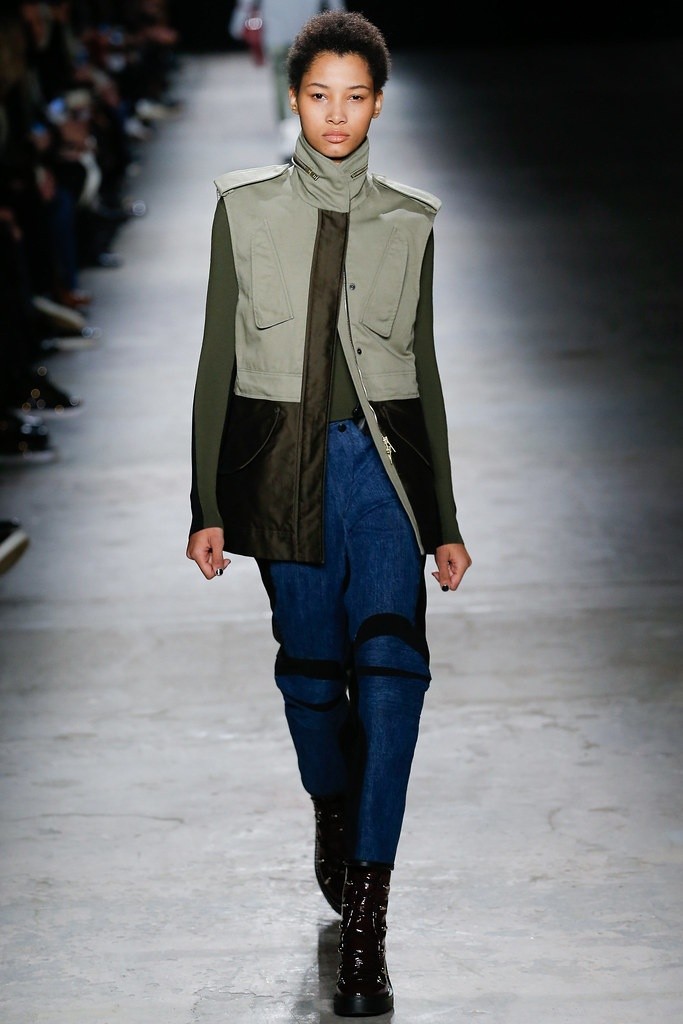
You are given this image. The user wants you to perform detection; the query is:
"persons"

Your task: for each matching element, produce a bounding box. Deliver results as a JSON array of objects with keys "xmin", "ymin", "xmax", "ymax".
[
  {"xmin": 186, "ymin": 8, "xmax": 471, "ymax": 1024},
  {"xmin": 0, "ymin": 0, "xmax": 247, "ymax": 575},
  {"xmin": 231, "ymin": 0, "xmax": 346, "ymax": 132}
]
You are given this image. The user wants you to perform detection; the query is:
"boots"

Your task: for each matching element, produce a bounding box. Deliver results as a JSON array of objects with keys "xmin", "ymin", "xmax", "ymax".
[
  {"xmin": 334, "ymin": 866, "xmax": 392, "ymax": 1016},
  {"xmin": 313, "ymin": 797, "xmax": 355, "ymax": 915}
]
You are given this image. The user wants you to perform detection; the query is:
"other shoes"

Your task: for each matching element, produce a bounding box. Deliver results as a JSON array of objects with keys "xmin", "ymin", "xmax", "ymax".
[
  {"xmin": 0, "ymin": 416, "xmax": 54, "ymax": 464},
  {"xmin": 0, "ymin": 520, "xmax": 30, "ymax": 579}
]
